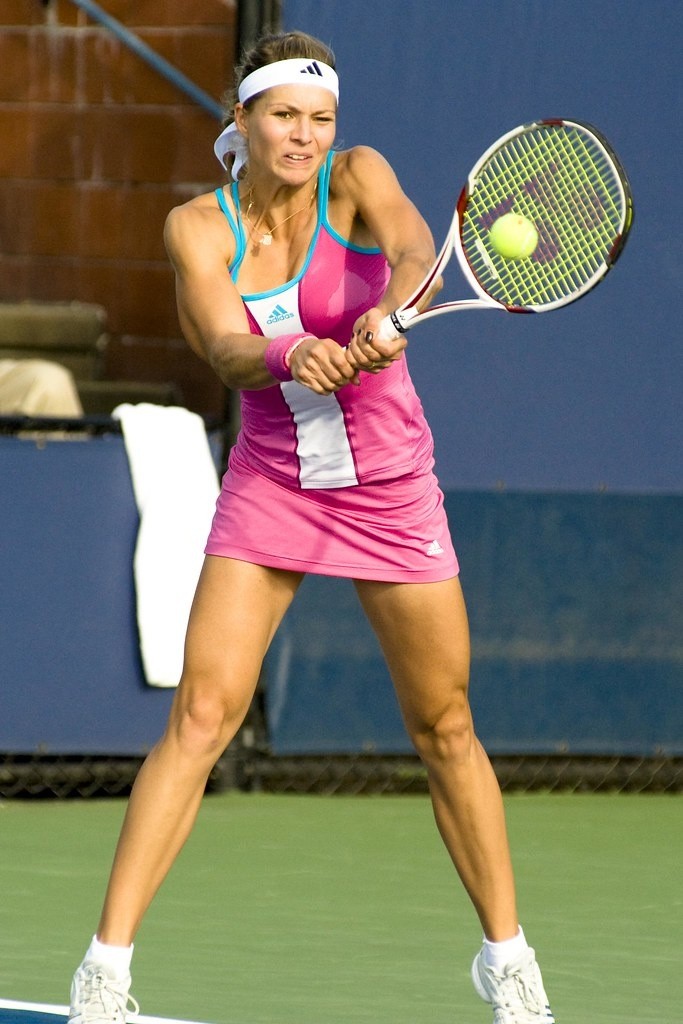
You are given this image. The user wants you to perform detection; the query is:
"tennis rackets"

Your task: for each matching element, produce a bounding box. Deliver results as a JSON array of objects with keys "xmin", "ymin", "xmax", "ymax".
[{"xmin": 342, "ymin": 114, "xmax": 638, "ymax": 353}]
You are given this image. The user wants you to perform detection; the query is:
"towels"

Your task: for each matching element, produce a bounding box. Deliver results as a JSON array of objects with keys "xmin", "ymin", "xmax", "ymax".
[{"xmin": 111, "ymin": 402, "xmax": 222, "ymax": 689}]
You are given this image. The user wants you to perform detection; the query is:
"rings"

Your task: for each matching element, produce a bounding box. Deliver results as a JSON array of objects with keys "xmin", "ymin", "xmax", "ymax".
[{"xmin": 368, "ymin": 361, "xmax": 376, "ymax": 369}]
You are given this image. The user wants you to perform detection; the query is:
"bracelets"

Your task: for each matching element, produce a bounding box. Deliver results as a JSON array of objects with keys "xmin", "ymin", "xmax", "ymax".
[
  {"xmin": 265, "ymin": 331, "xmax": 316, "ymax": 382},
  {"xmin": 284, "ymin": 336, "xmax": 318, "ymax": 368}
]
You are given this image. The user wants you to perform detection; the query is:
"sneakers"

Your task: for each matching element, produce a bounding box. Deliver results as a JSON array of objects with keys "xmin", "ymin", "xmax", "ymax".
[
  {"xmin": 67, "ymin": 946, "xmax": 139, "ymax": 1024},
  {"xmin": 470, "ymin": 944, "xmax": 557, "ymax": 1024}
]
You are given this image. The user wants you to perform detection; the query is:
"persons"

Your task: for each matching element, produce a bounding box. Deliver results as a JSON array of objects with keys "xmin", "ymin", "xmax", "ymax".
[{"xmin": 66, "ymin": 27, "xmax": 559, "ymax": 1024}]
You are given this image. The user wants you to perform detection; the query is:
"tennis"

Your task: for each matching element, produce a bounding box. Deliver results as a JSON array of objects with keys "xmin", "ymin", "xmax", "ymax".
[{"xmin": 489, "ymin": 213, "xmax": 539, "ymax": 260}]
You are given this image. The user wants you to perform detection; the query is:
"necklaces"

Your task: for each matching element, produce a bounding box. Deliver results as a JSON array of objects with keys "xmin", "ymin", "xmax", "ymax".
[{"xmin": 242, "ymin": 172, "xmax": 319, "ymax": 245}]
